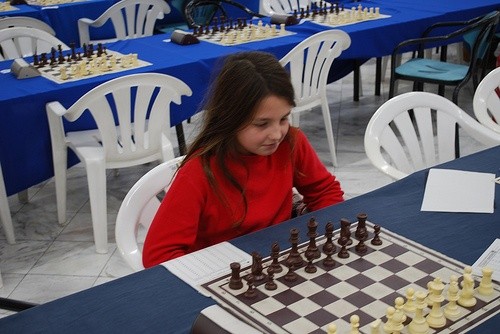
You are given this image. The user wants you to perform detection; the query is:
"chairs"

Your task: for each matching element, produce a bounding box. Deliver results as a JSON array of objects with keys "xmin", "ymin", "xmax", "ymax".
[{"xmin": 0, "ymin": 0, "xmax": 500, "ymax": 272}]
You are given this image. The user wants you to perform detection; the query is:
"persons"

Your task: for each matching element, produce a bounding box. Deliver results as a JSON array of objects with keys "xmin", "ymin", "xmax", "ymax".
[{"xmin": 142, "ymin": 51, "xmax": 344, "ymax": 269}]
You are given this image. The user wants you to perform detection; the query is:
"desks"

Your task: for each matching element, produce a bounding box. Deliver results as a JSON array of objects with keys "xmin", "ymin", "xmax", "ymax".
[
  {"xmin": 0, "ymin": 0, "xmax": 260, "ymax": 51},
  {"xmin": 0, "ymin": 0, "xmax": 500, "ymax": 206},
  {"xmin": 0, "ymin": 145, "xmax": 500, "ymax": 334}
]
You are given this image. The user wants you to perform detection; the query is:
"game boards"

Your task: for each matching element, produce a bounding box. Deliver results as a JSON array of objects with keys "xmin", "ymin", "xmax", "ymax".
[
  {"xmin": 198, "ymin": 219, "xmax": 499, "ymax": 334},
  {"xmin": 190, "ymin": 23, "xmax": 297, "ymax": 46},
  {"xmin": 29, "ymin": 49, "xmax": 153, "ymax": 85},
  {"xmin": 297, "ymin": 8, "xmax": 392, "ymax": 28}
]
